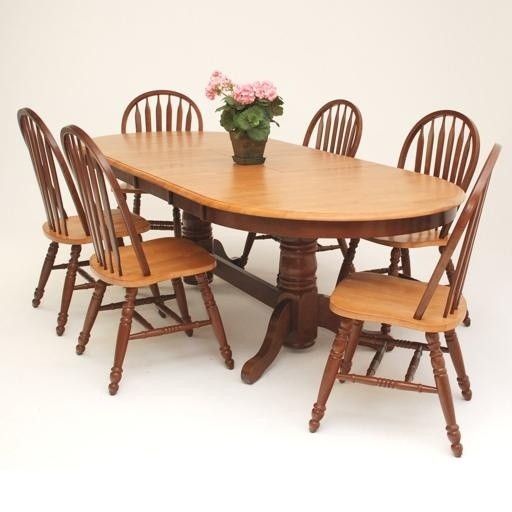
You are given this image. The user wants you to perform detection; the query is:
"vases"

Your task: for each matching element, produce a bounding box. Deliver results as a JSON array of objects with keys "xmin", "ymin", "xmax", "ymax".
[{"xmin": 230, "ymin": 134, "xmax": 267, "ymax": 165}]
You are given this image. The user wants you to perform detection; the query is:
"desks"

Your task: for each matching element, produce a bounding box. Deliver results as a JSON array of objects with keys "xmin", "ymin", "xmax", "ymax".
[{"xmin": 82, "ymin": 133, "xmax": 465, "ymax": 384}]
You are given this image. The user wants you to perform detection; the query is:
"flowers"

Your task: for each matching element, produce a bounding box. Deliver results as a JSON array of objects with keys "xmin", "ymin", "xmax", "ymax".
[{"xmin": 205, "ymin": 69, "xmax": 285, "ymax": 141}]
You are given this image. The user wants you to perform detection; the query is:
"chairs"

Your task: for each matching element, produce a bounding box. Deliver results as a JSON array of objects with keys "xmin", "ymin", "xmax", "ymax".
[
  {"xmin": 112, "ymin": 89, "xmax": 203, "ymax": 242},
  {"xmin": 61, "ymin": 125, "xmax": 234, "ymax": 396},
  {"xmin": 309, "ymin": 143, "xmax": 501, "ymax": 458},
  {"xmin": 336, "ymin": 110, "xmax": 480, "ymax": 327},
  {"xmin": 17, "ymin": 108, "xmax": 165, "ymax": 336},
  {"xmin": 241, "ymin": 99, "xmax": 362, "ymax": 274}
]
